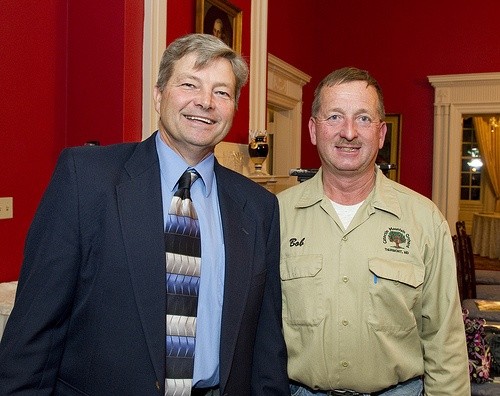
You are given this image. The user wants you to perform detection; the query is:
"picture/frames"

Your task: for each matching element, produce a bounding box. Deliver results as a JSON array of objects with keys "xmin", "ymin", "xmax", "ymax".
[
  {"xmin": 195, "ymin": 0, "xmax": 243, "ymax": 58},
  {"xmin": 375, "ymin": 113, "xmax": 402, "ymax": 184}
]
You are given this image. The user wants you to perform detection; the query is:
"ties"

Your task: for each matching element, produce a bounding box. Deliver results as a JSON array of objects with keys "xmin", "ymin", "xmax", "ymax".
[{"xmin": 164, "ymin": 168, "xmax": 201, "ymax": 396}]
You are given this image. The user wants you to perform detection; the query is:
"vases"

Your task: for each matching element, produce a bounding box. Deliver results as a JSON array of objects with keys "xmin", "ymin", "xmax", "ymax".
[{"xmin": 248, "ymin": 136, "xmax": 269, "ymax": 176}]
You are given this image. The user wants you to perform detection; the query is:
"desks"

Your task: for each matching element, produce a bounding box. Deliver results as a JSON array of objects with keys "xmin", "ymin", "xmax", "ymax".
[{"xmin": 470, "ymin": 212, "xmax": 500, "ymax": 259}]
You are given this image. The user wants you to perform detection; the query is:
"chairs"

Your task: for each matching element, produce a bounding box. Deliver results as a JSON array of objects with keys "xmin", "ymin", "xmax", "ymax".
[{"xmin": 450, "ymin": 220, "xmax": 500, "ymax": 362}]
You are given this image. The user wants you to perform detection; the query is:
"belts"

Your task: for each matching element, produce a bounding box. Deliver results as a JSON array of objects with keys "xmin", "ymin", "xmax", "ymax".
[{"xmin": 291, "ymin": 376, "xmax": 422, "ymax": 396}]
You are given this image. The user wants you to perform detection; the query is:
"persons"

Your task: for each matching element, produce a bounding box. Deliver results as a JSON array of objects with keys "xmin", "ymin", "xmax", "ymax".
[
  {"xmin": 276, "ymin": 67, "xmax": 471, "ymax": 396},
  {"xmin": 0, "ymin": 34, "xmax": 292, "ymax": 396}
]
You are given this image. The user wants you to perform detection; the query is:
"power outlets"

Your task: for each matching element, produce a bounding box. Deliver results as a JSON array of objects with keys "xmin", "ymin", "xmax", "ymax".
[{"xmin": 0, "ymin": 197, "xmax": 13, "ymax": 219}]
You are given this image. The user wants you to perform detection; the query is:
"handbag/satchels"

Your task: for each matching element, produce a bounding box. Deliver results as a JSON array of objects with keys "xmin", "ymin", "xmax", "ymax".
[{"xmin": 462, "ymin": 308, "xmax": 495, "ymax": 384}]
